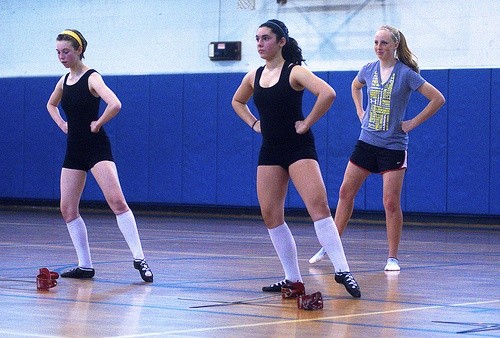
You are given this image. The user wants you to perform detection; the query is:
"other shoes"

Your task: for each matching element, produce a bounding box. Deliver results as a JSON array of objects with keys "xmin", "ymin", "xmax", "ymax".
[
  {"xmin": 61, "ymin": 266, "xmax": 95, "ymax": 279},
  {"xmin": 334, "ymin": 271, "xmax": 361, "ymax": 298},
  {"xmin": 309, "ymin": 247, "xmax": 328, "ymax": 264},
  {"xmin": 133, "ymin": 258, "xmax": 154, "ymax": 283},
  {"xmin": 262, "ymin": 279, "xmax": 292, "ymax": 292},
  {"xmin": 384, "ymin": 257, "xmax": 400, "ymax": 271}
]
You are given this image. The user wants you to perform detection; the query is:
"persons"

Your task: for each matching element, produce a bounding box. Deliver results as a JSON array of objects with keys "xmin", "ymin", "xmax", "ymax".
[
  {"xmin": 231, "ymin": 19, "xmax": 362, "ymax": 298},
  {"xmin": 310, "ymin": 25, "xmax": 444, "ymax": 271},
  {"xmin": 46, "ymin": 29, "xmax": 153, "ymax": 282}
]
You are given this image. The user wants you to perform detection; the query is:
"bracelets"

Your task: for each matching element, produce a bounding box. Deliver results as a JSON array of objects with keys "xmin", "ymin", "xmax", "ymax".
[{"xmin": 252, "ymin": 119, "xmax": 258, "ymax": 129}]
